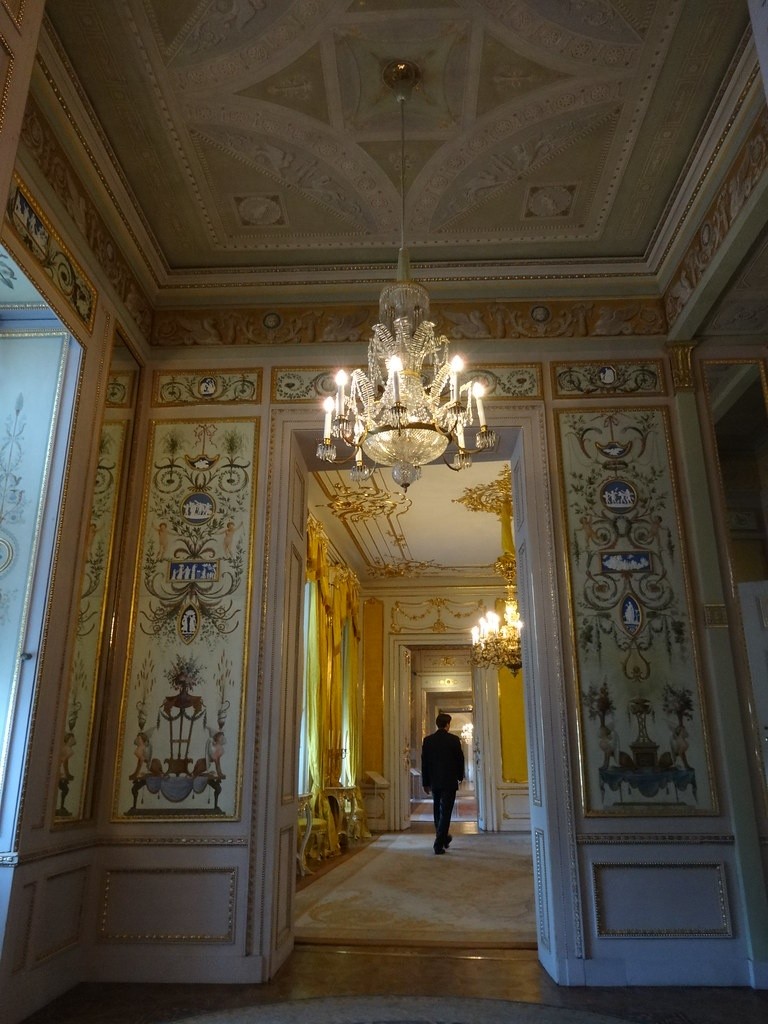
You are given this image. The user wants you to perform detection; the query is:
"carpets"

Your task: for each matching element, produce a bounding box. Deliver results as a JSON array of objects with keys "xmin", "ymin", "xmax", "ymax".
[
  {"xmin": 291, "ymin": 831, "xmax": 539, "ymax": 950},
  {"xmin": 409, "ymin": 795, "xmax": 478, "ymax": 822},
  {"xmin": 171, "ymin": 994, "xmax": 634, "ymax": 1023}
]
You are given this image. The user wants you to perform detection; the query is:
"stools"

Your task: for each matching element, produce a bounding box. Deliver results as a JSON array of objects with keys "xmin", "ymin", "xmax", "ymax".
[{"xmin": 298, "ymin": 817, "xmax": 328, "ymax": 863}]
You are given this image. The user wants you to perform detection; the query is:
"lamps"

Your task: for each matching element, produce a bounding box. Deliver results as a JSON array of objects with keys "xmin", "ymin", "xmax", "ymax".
[
  {"xmin": 314, "ymin": 55, "xmax": 500, "ymax": 494},
  {"xmin": 449, "ymin": 466, "xmax": 524, "ymax": 678}
]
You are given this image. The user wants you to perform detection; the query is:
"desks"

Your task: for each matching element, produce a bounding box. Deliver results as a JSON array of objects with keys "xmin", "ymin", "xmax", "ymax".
[
  {"xmin": 324, "ymin": 785, "xmax": 357, "ymax": 851},
  {"xmin": 296, "ymin": 793, "xmax": 313, "ymax": 877}
]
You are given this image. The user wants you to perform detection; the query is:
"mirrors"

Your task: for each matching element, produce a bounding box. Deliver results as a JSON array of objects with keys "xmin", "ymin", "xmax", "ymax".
[
  {"xmin": 693, "ymin": 343, "xmax": 768, "ymax": 883},
  {"xmin": 40, "ymin": 318, "xmax": 145, "ymax": 833}
]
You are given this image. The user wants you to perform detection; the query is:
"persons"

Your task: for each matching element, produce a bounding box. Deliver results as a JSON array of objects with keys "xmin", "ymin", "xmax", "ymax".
[{"xmin": 422, "ymin": 714, "xmax": 465, "ymax": 853}]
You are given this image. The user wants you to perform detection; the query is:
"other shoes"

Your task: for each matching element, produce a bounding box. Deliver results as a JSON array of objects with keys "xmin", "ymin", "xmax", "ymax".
[
  {"xmin": 444, "ymin": 835, "xmax": 452, "ymax": 849},
  {"xmin": 435, "ymin": 849, "xmax": 445, "ymax": 855}
]
